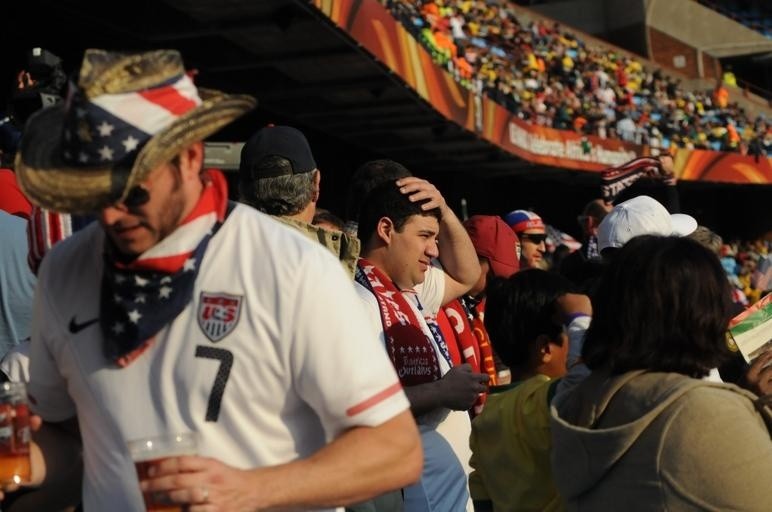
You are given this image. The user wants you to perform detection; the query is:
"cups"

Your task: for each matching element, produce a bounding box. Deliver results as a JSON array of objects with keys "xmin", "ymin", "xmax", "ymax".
[
  {"xmin": 125, "ymin": 430, "xmax": 207, "ymax": 511},
  {"xmin": 1, "ymin": 383, "xmax": 32, "ymax": 488}
]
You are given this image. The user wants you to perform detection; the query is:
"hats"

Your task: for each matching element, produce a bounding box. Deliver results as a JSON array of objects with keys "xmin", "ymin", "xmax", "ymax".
[
  {"xmin": 597, "ymin": 194, "xmax": 698, "ymax": 253},
  {"xmin": 14, "ymin": 48, "xmax": 260, "ymax": 218},
  {"xmin": 462, "ymin": 213, "xmax": 522, "ymax": 280},
  {"xmin": 239, "ymin": 123, "xmax": 317, "ymax": 184},
  {"xmin": 504, "ymin": 209, "xmax": 545, "ymax": 234}
]
